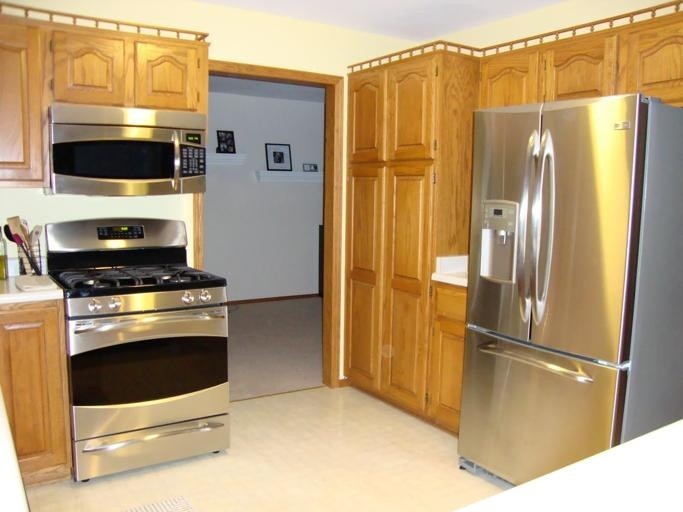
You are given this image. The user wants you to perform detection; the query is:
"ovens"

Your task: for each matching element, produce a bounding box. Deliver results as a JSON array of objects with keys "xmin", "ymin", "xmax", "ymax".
[{"xmin": 66, "ymin": 305, "xmax": 230, "ymax": 482}]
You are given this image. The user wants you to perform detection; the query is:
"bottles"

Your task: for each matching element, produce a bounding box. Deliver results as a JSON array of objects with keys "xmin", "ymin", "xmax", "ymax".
[{"xmin": 0, "ymin": 225, "xmax": 8, "ymax": 280}]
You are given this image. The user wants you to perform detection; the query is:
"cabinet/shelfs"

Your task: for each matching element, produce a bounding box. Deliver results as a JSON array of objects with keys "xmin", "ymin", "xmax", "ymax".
[
  {"xmin": 423, "ymin": 280, "xmax": 467, "ymax": 434},
  {"xmin": 51, "ymin": 13, "xmax": 199, "ymax": 111},
  {"xmin": 343, "ymin": 40, "xmax": 478, "ymax": 419},
  {"xmin": 478, "ymin": 1, "xmax": 682, "ymax": 109},
  {"xmin": 0, "ymin": 1, "xmax": 51, "ymax": 187},
  {"xmin": 1, "ymin": 300, "xmax": 72, "ymax": 489}
]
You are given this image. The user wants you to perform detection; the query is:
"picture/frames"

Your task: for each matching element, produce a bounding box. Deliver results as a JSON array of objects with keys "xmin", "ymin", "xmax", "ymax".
[
  {"xmin": 265, "ymin": 143, "xmax": 292, "ymax": 171},
  {"xmin": 217, "ymin": 130, "xmax": 236, "ymax": 153}
]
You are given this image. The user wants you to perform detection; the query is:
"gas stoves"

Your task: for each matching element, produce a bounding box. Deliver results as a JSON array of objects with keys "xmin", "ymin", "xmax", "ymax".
[{"xmin": 47, "ymin": 263, "xmax": 228, "ymax": 317}]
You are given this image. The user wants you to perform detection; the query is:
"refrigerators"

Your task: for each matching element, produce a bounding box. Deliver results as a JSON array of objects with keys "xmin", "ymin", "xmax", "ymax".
[{"xmin": 457, "ymin": 93, "xmax": 682, "ymax": 487}]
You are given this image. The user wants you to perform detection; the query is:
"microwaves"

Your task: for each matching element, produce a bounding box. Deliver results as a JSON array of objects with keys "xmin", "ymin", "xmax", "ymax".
[{"xmin": 47, "ymin": 102, "xmax": 207, "ymax": 197}]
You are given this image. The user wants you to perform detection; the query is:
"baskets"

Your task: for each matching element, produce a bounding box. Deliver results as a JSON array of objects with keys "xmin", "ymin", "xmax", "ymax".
[{"xmin": 17, "ymin": 238, "xmax": 41, "ymax": 276}]
